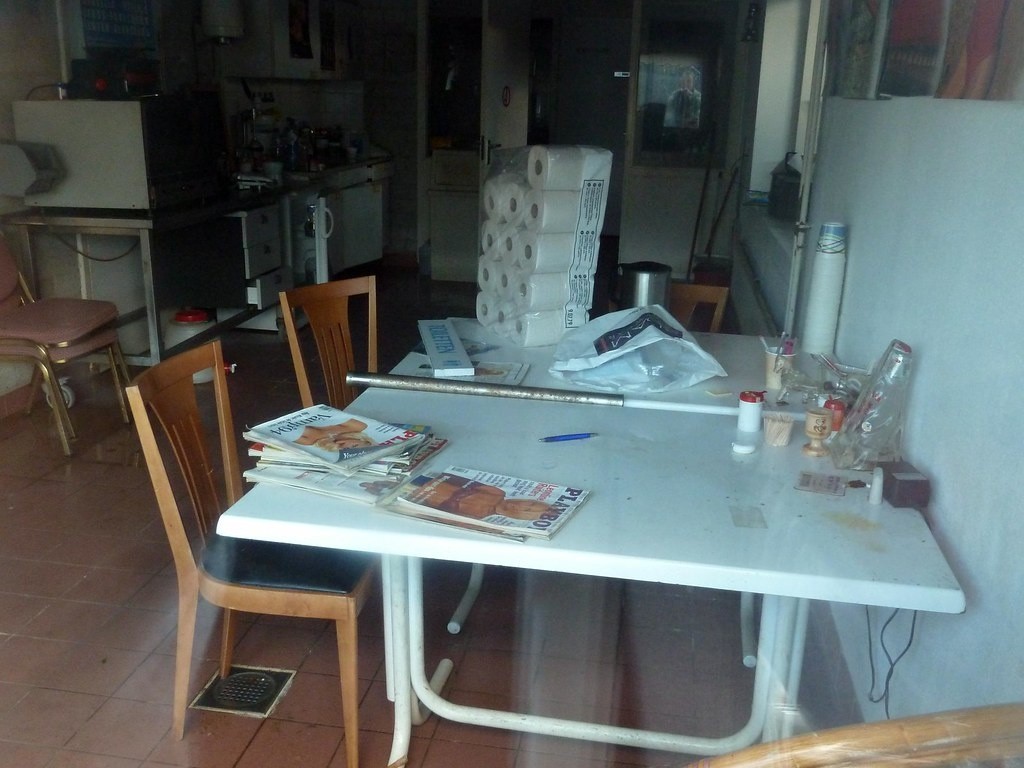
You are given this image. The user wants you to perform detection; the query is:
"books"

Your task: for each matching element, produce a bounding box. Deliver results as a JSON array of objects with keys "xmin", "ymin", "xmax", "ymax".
[
  {"xmin": 377, "ymin": 457, "xmax": 592, "ymax": 541},
  {"xmin": 239, "ymin": 402, "xmax": 450, "ymax": 504},
  {"xmin": 406, "ymin": 336, "xmax": 529, "ymax": 388}
]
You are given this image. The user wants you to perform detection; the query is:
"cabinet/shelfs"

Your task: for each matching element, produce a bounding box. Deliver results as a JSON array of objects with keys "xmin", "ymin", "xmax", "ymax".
[{"xmin": 224, "ymin": 0, "xmax": 350, "ymax": 80}]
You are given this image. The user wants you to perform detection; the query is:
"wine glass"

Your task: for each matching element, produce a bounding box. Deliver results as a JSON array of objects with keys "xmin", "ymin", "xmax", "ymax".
[{"xmin": 803, "ymin": 408, "xmax": 834, "ymax": 456}]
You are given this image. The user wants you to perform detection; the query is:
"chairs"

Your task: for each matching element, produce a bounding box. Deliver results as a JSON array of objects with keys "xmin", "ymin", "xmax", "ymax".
[
  {"xmin": 0, "ymin": 233, "xmax": 133, "ymax": 458},
  {"xmin": 123, "ymin": 335, "xmax": 374, "ymax": 768},
  {"xmin": 667, "ymin": 281, "xmax": 729, "ymax": 333},
  {"xmin": 279, "ymin": 275, "xmax": 384, "ymax": 412}
]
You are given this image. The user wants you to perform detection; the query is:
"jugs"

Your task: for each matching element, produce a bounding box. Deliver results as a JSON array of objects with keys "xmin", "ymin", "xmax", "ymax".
[{"xmin": 610, "ymin": 261, "xmax": 672, "ymax": 311}]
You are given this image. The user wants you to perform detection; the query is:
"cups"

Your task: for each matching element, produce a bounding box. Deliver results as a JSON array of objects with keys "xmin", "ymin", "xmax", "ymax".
[
  {"xmin": 802, "ymin": 223, "xmax": 846, "ymax": 356},
  {"xmin": 765, "ymin": 347, "xmax": 796, "ymax": 389},
  {"xmin": 818, "ymin": 394, "xmax": 841, "ymax": 407},
  {"xmin": 825, "ymin": 401, "xmax": 845, "ymax": 431},
  {"xmin": 765, "ymin": 415, "xmax": 794, "ymax": 446}
]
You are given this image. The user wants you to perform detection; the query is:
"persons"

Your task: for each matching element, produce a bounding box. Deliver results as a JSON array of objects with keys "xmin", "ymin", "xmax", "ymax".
[
  {"xmin": 403, "ymin": 472, "xmax": 558, "ymax": 522},
  {"xmin": 667, "ymin": 71, "xmax": 701, "ymax": 130},
  {"xmin": 293, "ymin": 420, "xmax": 377, "ymax": 452},
  {"xmin": 358, "ymin": 479, "xmax": 400, "ymax": 499},
  {"xmin": 474, "ymin": 365, "xmax": 506, "ymax": 379}
]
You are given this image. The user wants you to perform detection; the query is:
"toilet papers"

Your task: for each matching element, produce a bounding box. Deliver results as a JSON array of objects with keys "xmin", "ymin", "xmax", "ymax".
[{"xmin": 471, "ymin": 140, "xmax": 615, "ymax": 345}]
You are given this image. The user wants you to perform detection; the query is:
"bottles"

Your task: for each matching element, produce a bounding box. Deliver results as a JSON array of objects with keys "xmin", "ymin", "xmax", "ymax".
[
  {"xmin": 270, "ymin": 128, "xmax": 285, "ymax": 160},
  {"xmin": 315, "ymin": 127, "xmax": 330, "ymax": 157},
  {"xmin": 737, "ymin": 391, "xmax": 763, "ymax": 432},
  {"xmin": 164, "ymin": 310, "xmax": 217, "ymax": 384},
  {"xmin": 299, "ymin": 128, "xmax": 313, "ymax": 163}
]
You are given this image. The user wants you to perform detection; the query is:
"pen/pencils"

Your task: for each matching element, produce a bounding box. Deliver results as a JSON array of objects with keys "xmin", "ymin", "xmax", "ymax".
[
  {"xmin": 538, "ymin": 432, "xmax": 599, "ymax": 443},
  {"xmin": 810, "ymin": 352, "xmax": 842, "ymax": 376},
  {"xmin": 758, "ymin": 330, "xmax": 800, "ymax": 355}
]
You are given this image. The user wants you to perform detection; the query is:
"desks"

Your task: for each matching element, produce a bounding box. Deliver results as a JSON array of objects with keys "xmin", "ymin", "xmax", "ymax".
[
  {"xmin": 0, "ymin": 207, "xmax": 293, "ymax": 368},
  {"xmin": 215, "ymin": 317, "xmax": 967, "ymax": 768}
]
